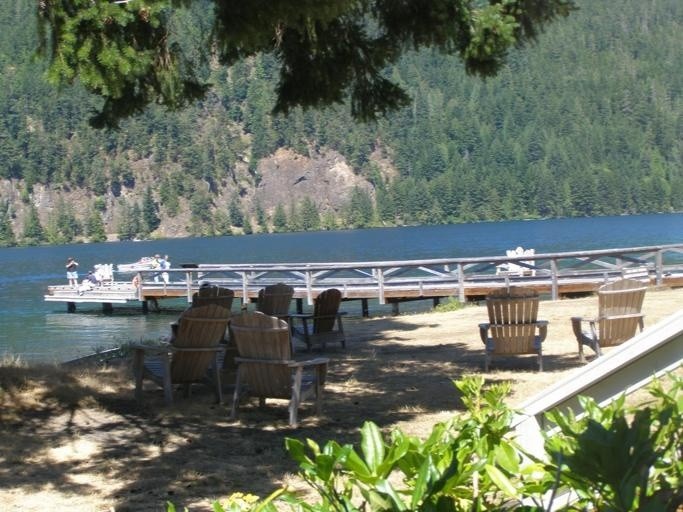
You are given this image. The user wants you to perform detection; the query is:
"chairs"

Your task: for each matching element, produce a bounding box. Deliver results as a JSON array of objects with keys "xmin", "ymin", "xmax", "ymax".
[
  {"xmin": 130, "ymin": 282, "xmax": 348, "ymax": 429},
  {"xmin": 475, "ymin": 245, "xmax": 649, "ymax": 374}
]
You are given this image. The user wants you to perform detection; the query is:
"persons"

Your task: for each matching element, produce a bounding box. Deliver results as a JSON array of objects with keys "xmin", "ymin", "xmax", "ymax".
[
  {"xmin": 133, "ymin": 272, "xmax": 143, "ymax": 298},
  {"xmin": 151, "ymin": 254, "xmax": 161, "ymax": 283},
  {"xmin": 65, "ymin": 258, "xmax": 78, "ymax": 289},
  {"xmin": 161, "ymin": 255, "xmax": 170, "ymax": 283},
  {"xmin": 86, "ymin": 270, "xmax": 97, "ymax": 287},
  {"xmin": 79, "ymin": 276, "xmax": 96, "ymax": 296}
]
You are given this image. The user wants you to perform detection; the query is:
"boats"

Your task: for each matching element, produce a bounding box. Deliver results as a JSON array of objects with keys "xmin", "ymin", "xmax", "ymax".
[{"xmin": 115, "ymin": 255, "xmax": 172, "ymax": 276}]
